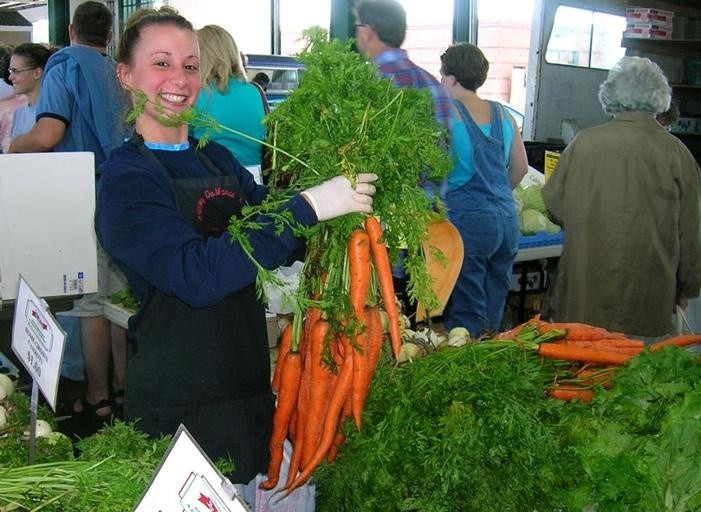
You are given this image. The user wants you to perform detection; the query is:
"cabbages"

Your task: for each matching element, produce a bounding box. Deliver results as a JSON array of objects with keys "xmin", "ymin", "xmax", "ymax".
[{"xmin": 512, "ymin": 184, "xmax": 561, "ymax": 236}]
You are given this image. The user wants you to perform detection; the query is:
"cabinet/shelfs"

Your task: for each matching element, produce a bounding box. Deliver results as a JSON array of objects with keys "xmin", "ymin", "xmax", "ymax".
[{"xmin": 617, "ymin": 34, "xmax": 701, "ymax": 143}]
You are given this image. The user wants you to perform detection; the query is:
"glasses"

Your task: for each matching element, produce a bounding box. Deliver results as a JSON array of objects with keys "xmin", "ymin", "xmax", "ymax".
[{"xmin": 8, "ymin": 63, "xmax": 40, "ymax": 75}]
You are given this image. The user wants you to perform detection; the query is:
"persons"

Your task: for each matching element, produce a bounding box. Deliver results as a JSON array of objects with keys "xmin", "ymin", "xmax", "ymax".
[
  {"xmin": 5, "ymin": 40, "xmax": 62, "ymax": 151},
  {"xmin": 542, "ymin": 53, "xmax": 700, "ymax": 337},
  {"xmin": 340, "ymin": 1, "xmax": 453, "ymax": 337},
  {"xmin": 435, "ymin": 35, "xmax": 530, "ymax": 340},
  {"xmin": 183, "ymin": 24, "xmax": 271, "ymax": 186},
  {"xmin": 91, "ymin": 3, "xmax": 379, "ymax": 512},
  {"xmin": 1, "ymin": 41, "xmax": 29, "ymax": 151},
  {"xmin": 7, "ymin": 0, "xmax": 128, "ymax": 425}
]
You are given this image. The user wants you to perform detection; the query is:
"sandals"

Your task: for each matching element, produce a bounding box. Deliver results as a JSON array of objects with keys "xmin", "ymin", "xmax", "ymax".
[{"xmin": 59, "ymin": 391, "xmax": 113, "ymax": 427}]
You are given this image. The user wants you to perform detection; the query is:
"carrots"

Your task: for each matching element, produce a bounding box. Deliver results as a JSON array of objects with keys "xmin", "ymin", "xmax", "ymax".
[
  {"xmin": 364, "ymin": 215, "xmax": 400, "ymax": 362},
  {"xmin": 259, "ymin": 224, "xmax": 382, "ymax": 505},
  {"xmin": 494, "ymin": 314, "xmax": 701, "ymax": 402}
]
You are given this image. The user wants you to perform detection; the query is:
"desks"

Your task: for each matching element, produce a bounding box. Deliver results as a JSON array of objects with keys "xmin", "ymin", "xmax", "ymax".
[{"xmin": 507, "ymin": 230, "xmax": 566, "ymax": 324}]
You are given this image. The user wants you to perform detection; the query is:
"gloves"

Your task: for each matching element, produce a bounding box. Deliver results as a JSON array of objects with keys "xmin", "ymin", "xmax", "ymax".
[{"xmin": 299, "ymin": 174, "xmax": 379, "ymax": 222}]
